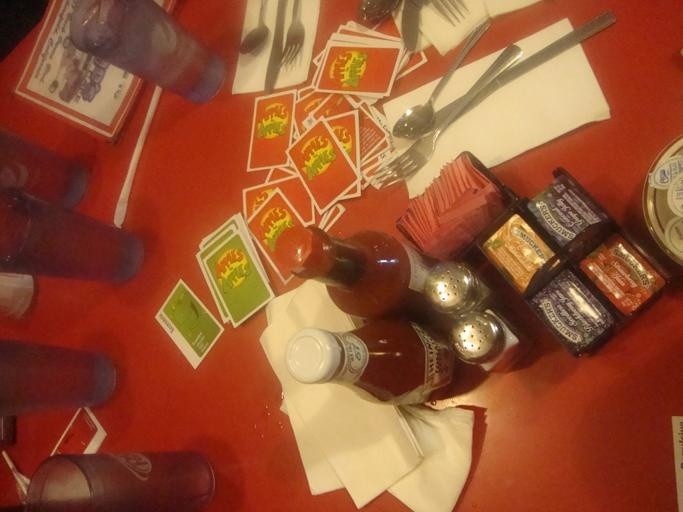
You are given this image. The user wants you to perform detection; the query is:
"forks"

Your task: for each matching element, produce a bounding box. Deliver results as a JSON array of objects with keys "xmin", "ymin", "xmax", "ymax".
[
  {"xmin": 371, "ymin": 42, "xmax": 523, "ymax": 187},
  {"xmin": 430, "ymin": 0, "xmax": 469, "ymax": 27},
  {"xmin": 279, "ymin": 0, "xmax": 304, "ymax": 67}
]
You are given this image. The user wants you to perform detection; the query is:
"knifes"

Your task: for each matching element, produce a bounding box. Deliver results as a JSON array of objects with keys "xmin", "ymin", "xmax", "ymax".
[
  {"xmin": 419, "ymin": 10, "xmax": 616, "ymax": 134},
  {"xmin": 263, "ymin": 0, "xmax": 287, "ymax": 94}
]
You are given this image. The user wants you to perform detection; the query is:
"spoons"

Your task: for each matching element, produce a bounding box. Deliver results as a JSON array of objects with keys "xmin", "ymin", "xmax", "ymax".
[
  {"xmin": 239, "ymin": 0, "xmax": 269, "ymax": 55},
  {"xmin": 390, "ymin": 16, "xmax": 492, "ymax": 139},
  {"xmin": 359, "ymin": 0, "xmax": 400, "ymax": 24}
]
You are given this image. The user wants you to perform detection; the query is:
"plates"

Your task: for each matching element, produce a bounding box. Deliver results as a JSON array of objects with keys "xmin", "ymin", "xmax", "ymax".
[{"xmin": 641, "ymin": 131, "xmax": 682, "ymax": 267}]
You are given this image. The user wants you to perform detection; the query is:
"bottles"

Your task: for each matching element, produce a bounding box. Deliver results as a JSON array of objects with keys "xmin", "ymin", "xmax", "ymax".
[
  {"xmin": 449, "ymin": 312, "xmax": 505, "ymax": 366},
  {"xmin": 424, "ymin": 260, "xmax": 480, "ymax": 315},
  {"xmin": 272, "ymin": 223, "xmax": 433, "ymax": 319},
  {"xmin": 282, "ymin": 325, "xmax": 459, "ymax": 406}
]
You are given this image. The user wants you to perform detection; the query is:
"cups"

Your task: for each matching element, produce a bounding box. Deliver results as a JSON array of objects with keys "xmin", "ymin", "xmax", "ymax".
[
  {"xmin": 25, "ymin": 450, "xmax": 215, "ymax": 512},
  {"xmin": 68, "ymin": 0, "xmax": 228, "ymax": 105},
  {"xmin": 0, "ymin": 131, "xmax": 88, "ymax": 210},
  {"xmin": 0, "ymin": 187, "xmax": 144, "ymax": 283},
  {"xmin": 0, "ymin": 339, "xmax": 117, "ymax": 419}
]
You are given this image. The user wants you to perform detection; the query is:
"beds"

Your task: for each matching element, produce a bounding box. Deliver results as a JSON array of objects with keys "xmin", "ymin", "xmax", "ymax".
[{"xmin": 1, "ymin": 0, "xmax": 682, "ymax": 511}]
